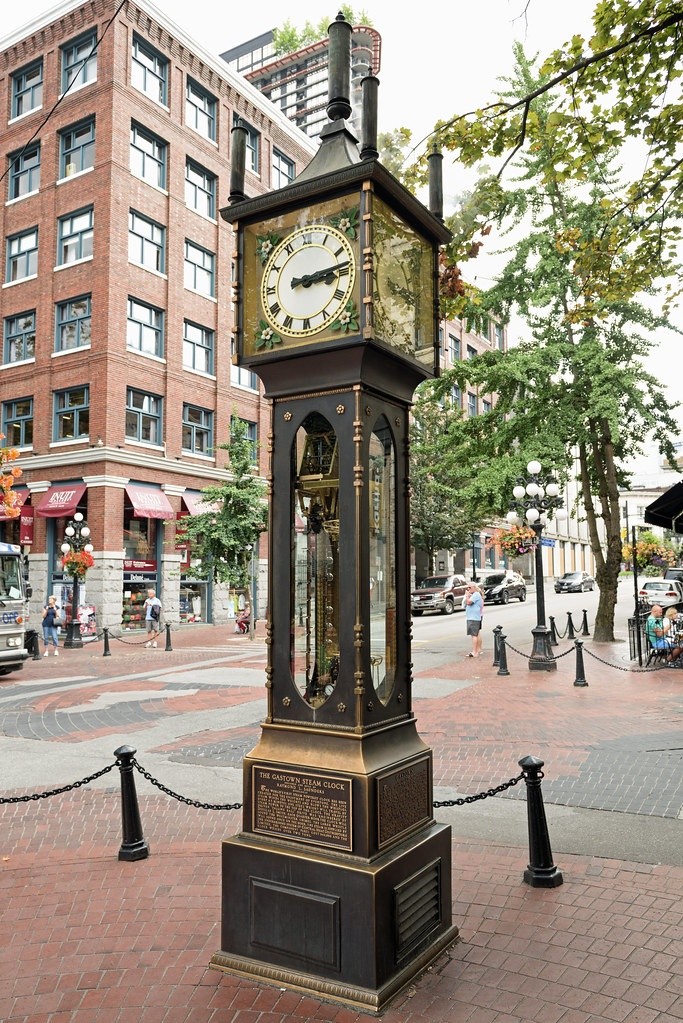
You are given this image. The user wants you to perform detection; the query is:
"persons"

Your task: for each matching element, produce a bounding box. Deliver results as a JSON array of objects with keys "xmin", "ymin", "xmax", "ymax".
[
  {"xmin": 142, "ymin": 590, "xmax": 162, "ymax": 648},
  {"xmin": 646, "ymin": 605, "xmax": 682, "ymax": 666},
  {"xmin": 236, "ymin": 601, "xmax": 251, "ymax": 634},
  {"xmin": 465, "ymin": 582, "xmax": 485, "ymax": 657},
  {"xmin": 41, "ymin": 595, "xmax": 60, "ymax": 657}
]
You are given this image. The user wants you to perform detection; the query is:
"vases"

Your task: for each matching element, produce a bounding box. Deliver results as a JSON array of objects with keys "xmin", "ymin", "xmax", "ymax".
[{"xmin": 67, "ymin": 564, "xmax": 78, "ymax": 570}]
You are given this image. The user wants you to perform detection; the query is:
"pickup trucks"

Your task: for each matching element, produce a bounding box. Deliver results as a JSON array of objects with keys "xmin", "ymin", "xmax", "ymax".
[{"xmin": 411, "ymin": 574, "xmax": 486, "ymax": 617}]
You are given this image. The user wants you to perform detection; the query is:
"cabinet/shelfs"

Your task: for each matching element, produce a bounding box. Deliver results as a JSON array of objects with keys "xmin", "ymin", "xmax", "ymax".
[{"xmin": 180, "ymin": 591, "xmax": 201, "ymax": 623}]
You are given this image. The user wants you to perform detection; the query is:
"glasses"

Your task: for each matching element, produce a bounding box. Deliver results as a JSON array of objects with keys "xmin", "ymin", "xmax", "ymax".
[{"xmin": 467, "ymin": 587, "xmax": 475, "ymax": 589}]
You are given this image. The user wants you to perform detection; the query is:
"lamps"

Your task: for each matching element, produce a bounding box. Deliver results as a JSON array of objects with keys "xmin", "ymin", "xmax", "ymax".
[
  {"xmin": 90, "ymin": 445, "xmax": 96, "ymax": 448},
  {"xmin": 117, "ymin": 446, "xmax": 125, "ymax": 449},
  {"xmin": 175, "ymin": 457, "xmax": 182, "ymax": 461},
  {"xmin": 32, "ymin": 453, "xmax": 39, "ymax": 456},
  {"xmin": 97, "ymin": 439, "xmax": 104, "ymax": 447}
]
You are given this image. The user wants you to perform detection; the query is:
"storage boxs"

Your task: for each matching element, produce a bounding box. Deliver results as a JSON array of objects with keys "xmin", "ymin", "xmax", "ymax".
[
  {"xmin": 65, "ymin": 163, "xmax": 76, "ymax": 176},
  {"xmin": 122, "ymin": 593, "xmax": 147, "ymax": 629}
]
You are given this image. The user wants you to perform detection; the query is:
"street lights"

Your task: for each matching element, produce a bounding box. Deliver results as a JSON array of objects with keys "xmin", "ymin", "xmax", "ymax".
[
  {"xmin": 506, "ymin": 461, "xmax": 568, "ymax": 671},
  {"xmin": 61, "ymin": 513, "xmax": 93, "ymax": 649}
]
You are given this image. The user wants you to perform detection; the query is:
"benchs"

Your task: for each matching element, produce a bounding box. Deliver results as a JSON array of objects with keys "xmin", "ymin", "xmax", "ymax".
[{"xmin": 236, "ymin": 619, "xmax": 260, "ymax": 633}]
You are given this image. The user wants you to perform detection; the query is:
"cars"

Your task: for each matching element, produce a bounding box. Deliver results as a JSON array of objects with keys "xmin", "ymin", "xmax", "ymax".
[
  {"xmin": 638, "ymin": 580, "xmax": 683, "ymax": 606},
  {"xmin": 554, "ymin": 571, "xmax": 595, "ymax": 593}
]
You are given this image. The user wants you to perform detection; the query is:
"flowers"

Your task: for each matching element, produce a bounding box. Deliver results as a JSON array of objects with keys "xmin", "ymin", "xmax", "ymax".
[
  {"xmin": 330, "ymin": 298, "xmax": 360, "ymax": 333},
  {"xmin": 483, "ymin": 524, "xmax": 539, "ymax": 562},
  {"xmin": 329, "ymin": 205, "xmax": 360, "ymax": 240},
  {"xmin": 254, "ymin": 319, "xmax": 282, "ymax": 349},
  {"xmin": 60, "ymin": 547, "xmax": 96, "ymax": 575},
  {"xmin": 254, "ymin": 230, "xmax": 280, "ymax": 266}
]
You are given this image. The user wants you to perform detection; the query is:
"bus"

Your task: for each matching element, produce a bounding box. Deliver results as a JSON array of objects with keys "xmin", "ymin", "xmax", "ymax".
[{"xmin": 0, "ymin": 543, "xmax": 34, "ymax": 675}]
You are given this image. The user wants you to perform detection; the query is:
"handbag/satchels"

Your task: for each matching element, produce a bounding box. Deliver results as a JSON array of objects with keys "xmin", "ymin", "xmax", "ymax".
[
  {"xmin": 54, "ymin": 614, "xmax": 62, "ymax": 626},
  {"xmin": 462, "ymin": 594, "xmax": 468, "ymax": 608},
  {"xmin": 234, "ymin": 623, "xmax": 240, "ymax": 634}
]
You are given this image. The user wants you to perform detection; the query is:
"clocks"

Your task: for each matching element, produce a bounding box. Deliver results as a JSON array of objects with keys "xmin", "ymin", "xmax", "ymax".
[
  {"xmin": 372, "ymin": 232, "xmax": 418, "ymax": 338},
  {"xmin": 260, "ymin": 224, "xmax": 356, "ymax": 338}
]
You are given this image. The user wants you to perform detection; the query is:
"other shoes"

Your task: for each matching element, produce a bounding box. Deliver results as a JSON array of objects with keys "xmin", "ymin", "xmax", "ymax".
[
  {"xmin": 144, "ymin": 644, "xmax": 152, "ymax": 648},
  {"xmin": 663, "ymin": 659, "xmax": 679, "ymax": 668},
  {"xmin": 44, "ymin": 651, "xmax": 48, "ymax": 656},
  {"xmin": 153, "ymin": 643, "xmax": 159, "ymax": 648},
  {"xmin": 54, "ymin": 650, "xmax": 58, "ymax": 656},
  {"xmin": 466, "ymin": 652, "xmax": 479, "ymax": 657}
]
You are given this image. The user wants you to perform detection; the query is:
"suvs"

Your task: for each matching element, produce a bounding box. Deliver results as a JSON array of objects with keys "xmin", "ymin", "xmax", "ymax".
[
  {"xmin": 484, "ymin": 571, "xmax": 527, "ymax": 605},
  {"xmin": 664, "ymin": 568, "xmax": 683, "ymax": 582}
]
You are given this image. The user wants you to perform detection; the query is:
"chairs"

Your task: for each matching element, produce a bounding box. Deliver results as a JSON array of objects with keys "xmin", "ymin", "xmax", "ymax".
[{"xmin": 643, "ymin": 631, "xmax": 683, "ymax": 668}]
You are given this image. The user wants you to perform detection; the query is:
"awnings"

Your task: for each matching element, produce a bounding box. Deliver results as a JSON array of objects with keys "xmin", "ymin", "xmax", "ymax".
[
  {"xmin": 0, "ymin": 490, "xmax": 29, "ymax": 521},
  {"xmin": 182, "ymin": 490, "xmax": 222, "ymax": 516},
  {"xmin": 125, "ymin": 482, "xmax": 174, "ymax": 519},
  {"xmin": 35, "ymin": 483, "xmax": 87, "ymax": 518},
  {"xmin": 644, "ymin": 480, "xmax": 683, "ymax": 535}
]
